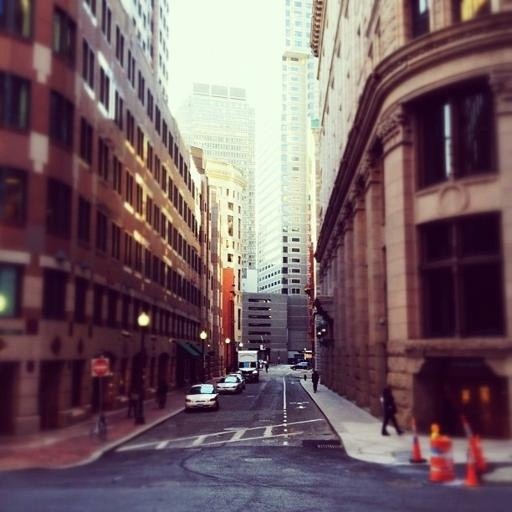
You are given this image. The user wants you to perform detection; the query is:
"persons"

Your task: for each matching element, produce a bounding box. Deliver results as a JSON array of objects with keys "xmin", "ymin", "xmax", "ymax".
[
  {"xmin": 154, "ymin": 380, "xmax": 171, "ymax": 410},
  {"xmin": 265, "ymin": 363, "xmax": 269, "ymax": 373},
  {"xmin": 382, "ymin": 387, "xmax": 403, "ymax": 437},
  {"xmin": 309, "ymin": 370, "xmax": 319, "ymax": 393}
]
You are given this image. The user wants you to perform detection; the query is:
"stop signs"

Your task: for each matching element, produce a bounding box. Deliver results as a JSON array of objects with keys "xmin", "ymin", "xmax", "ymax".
[{"xmin": 91, "ymin": 359, "xmax": 110, "ymax": 378}]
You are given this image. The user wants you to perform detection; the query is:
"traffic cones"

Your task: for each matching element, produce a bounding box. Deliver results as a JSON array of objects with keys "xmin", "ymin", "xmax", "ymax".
[
  {"xmin": 410, "ymin": 419, "xmax": 425, "ymax": 464},
  {"xmin": 462, "ymin": 418, "xmax": 488, "ymax": 487}
]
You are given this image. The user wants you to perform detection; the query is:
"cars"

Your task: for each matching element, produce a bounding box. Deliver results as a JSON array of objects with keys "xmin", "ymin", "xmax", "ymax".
[
  {"xmin": 290, "ymin": 362, "xmax": 309, "ymax": 370},
  {"xmin": 185, "ymin": 384, "xmax": 219, "ymax": 413},
  {"xmin": 216, "ymin": 374, "xmax": 246, "ymax": 394}
]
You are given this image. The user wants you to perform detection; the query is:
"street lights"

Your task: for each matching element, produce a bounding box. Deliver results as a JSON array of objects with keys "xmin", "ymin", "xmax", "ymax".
[
  {"xmin": 200, "ymin": 331, "xmax": 207, "ymax": 380},
  {"xmin": 225, "ymin": 338, "xmax": 231, "ymax": 368},
  {"xmin": 137, "ymin": 311, "xmax": 150, "ymax": 424}
]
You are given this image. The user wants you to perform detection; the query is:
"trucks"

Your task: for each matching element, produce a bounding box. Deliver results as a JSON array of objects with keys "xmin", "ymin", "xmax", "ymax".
[{"xmin": 237, "ymin": 351, "xmax": 259, "ymax": 380}]
